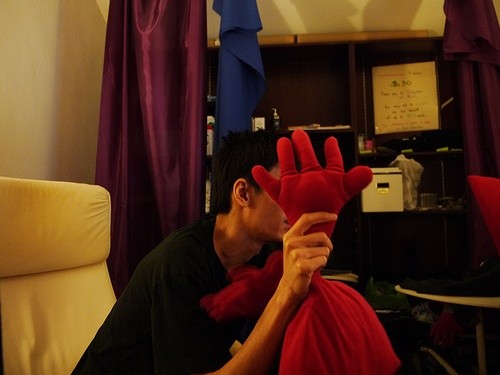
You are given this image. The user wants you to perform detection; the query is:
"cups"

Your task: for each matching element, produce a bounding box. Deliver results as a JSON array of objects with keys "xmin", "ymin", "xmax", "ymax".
[{"xmin": 421, "ymin": 194, "xmax": 437, "ymax": 211}]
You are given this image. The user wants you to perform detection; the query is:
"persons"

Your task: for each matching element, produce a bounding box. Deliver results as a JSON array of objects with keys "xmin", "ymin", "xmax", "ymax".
[{"xmin": 69, "ymin": 127, "xmax": 339, "ymax": 375}]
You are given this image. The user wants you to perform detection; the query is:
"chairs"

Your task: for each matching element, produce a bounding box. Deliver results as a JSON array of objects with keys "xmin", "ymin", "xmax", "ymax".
[
  {"xmin": 0, "ymin": 177, "xmax": 117, "ymax": 375},
  {"xmin": 467, "ymin": 174, "xmax": 499, "ymax": 252}
]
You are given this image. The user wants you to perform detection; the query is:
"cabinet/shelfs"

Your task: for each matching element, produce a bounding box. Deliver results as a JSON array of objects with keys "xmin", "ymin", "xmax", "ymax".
[{"xmin": 192, "ymin": 27, "xmax": 467, "ymax": 231}]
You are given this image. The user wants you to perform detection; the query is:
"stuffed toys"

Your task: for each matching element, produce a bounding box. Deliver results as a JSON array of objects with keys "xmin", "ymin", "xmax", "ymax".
[{"xmin": 199, "ymin": 129, "xmax": 401, "ymax": 375}]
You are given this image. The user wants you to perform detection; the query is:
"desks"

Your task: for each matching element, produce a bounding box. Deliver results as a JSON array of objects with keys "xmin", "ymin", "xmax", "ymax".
[{"xmin": 394, "ymin": 272, "xmax": 500, "ymax": 374}]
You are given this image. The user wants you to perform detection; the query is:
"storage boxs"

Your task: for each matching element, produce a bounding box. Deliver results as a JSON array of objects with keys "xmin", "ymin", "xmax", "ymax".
[{"xmin": 361, "ymin": 166, "xmax": 404, "ymax": 213}]
